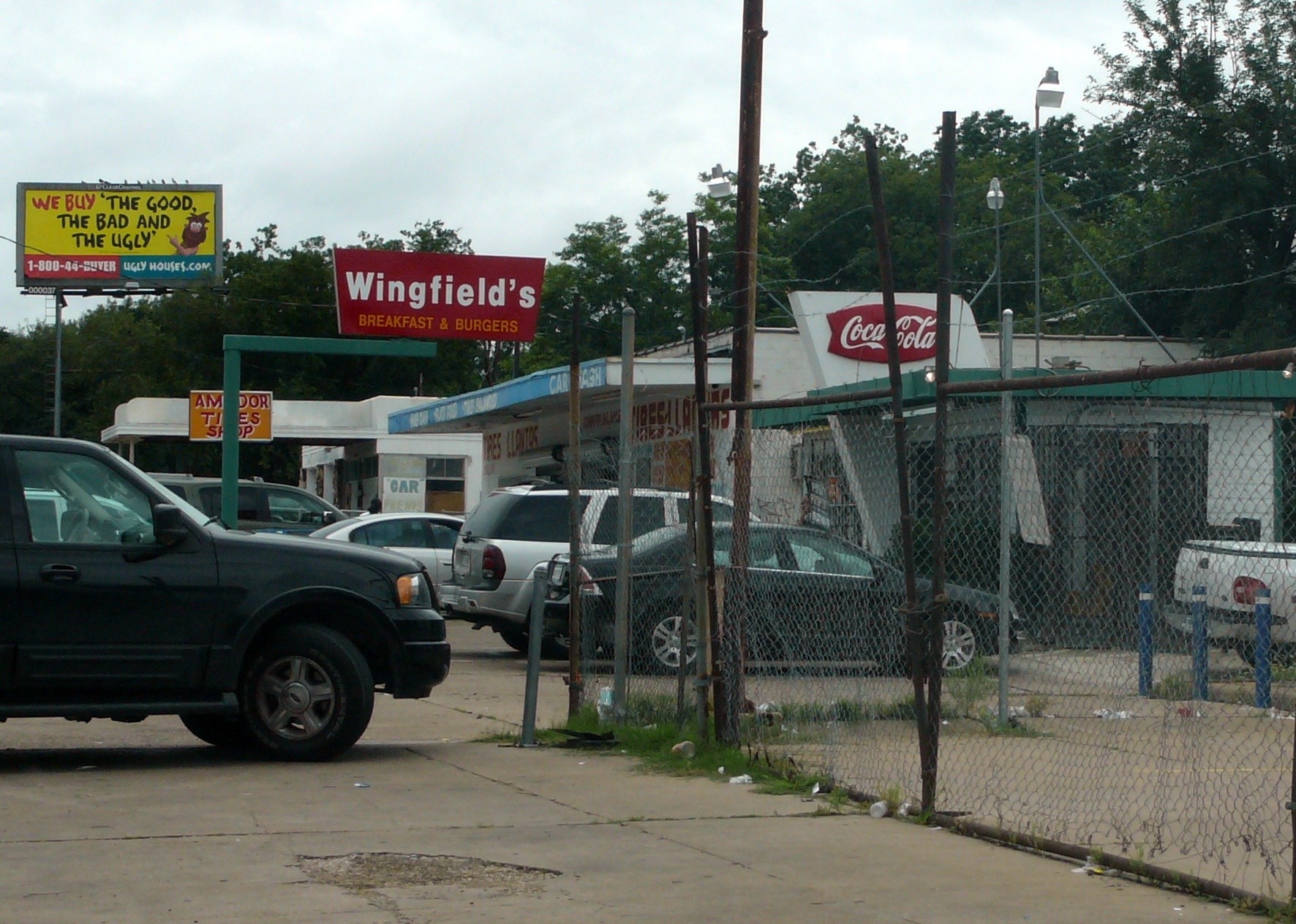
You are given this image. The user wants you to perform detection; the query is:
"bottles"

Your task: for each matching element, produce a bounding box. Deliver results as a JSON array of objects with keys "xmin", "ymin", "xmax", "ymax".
[{"xmin": 869, "ymin": 799, "xmax": 890, "ymax": 818}]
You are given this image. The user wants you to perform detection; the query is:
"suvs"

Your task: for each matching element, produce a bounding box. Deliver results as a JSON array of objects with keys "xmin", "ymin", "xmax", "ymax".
[
  {"xmin": 434, "ymin": 475, "xmax": 875, "ymax": 662},
  {"xmin": 21, "ymin": 483, "xmax": 156, "ymax": 545},
  {"xmin": 0, "ymin": 434, "xmax": 453, "ymax": 763},
  {"xmin": 132, "ymin": 470, "xmax": 356, "ymax": 535}
]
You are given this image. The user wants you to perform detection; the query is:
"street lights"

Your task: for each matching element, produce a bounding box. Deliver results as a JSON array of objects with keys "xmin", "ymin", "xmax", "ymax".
[{"xmin": 1033, "ymin": 65, "xmax": 1065, "ymax": 372}]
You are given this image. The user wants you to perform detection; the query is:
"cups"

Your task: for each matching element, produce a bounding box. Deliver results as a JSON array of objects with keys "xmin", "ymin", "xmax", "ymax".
[{"xmin": 672, "ymin": 740, "xmax": 695, "ymax": 758}]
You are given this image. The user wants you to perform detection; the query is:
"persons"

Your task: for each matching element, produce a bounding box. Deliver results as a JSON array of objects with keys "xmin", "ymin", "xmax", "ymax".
[{"xmin": 367, "ymin": 494, "xmax": 382, "ymax": 514}]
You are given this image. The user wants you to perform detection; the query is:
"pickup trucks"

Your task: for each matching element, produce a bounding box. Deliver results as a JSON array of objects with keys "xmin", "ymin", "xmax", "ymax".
[{"xmin": 1156, "ymin": 537, "xmax": 1296, "ymax": 672}]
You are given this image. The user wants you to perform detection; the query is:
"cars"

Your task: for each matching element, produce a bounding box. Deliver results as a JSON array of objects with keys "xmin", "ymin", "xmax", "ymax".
[
  {"xmin": 540, "ymin": 520, "xmax": 1027, "ymax": 680},
  {"xmin": 307, "ymin": 511, "xmax": 467, "ymax": 617}
]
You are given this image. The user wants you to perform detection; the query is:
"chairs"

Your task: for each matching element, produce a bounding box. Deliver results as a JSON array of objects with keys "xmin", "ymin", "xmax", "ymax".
[{"xmin": 754, "ymin": 541, "xmax": 774, "ymax": 569}]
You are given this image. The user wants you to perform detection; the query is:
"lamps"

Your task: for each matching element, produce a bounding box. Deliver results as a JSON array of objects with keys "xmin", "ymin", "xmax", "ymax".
[
  {"xmin": 924, "ymin": 365, "xmax": 936, "ymax": 382},
  {"xmin": 1283, "ymin": 362, "xmax": 1294, "ymax": 378}
]
